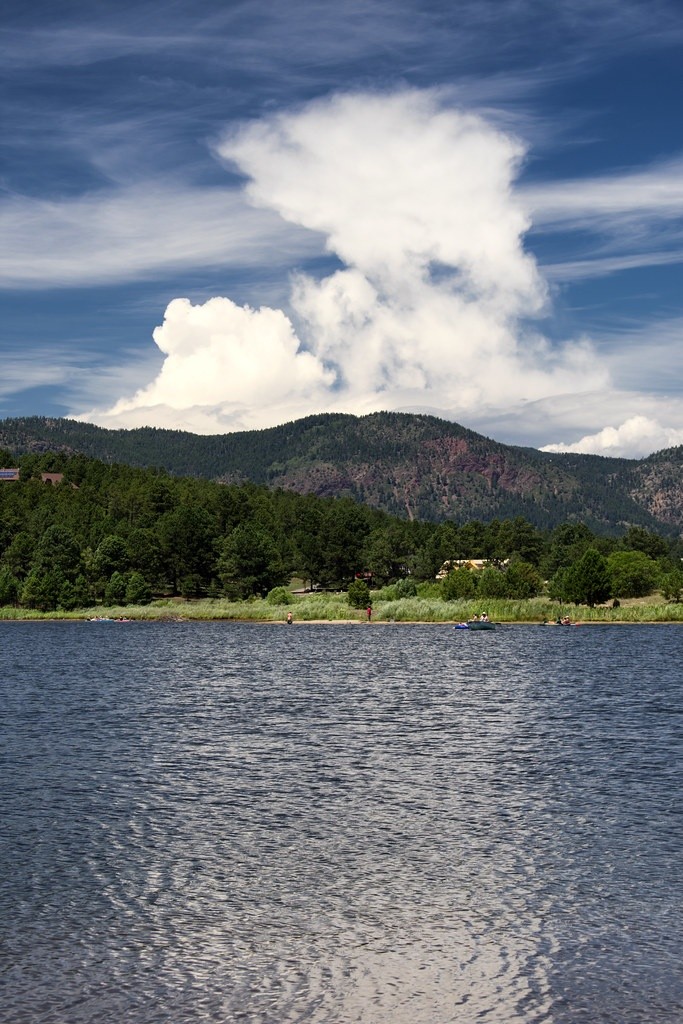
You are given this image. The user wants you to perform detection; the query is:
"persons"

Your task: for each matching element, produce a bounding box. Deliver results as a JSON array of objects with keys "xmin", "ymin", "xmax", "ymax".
[
  {"xmin": 481, "ymin": 612, "xmax": 489, "ymax": 623},
  {"xmin": 287, "ymin": 612, "xmax": 292, "ymax": 624},
  {"xmin": 366, "ymin": 605, "xmax": 371, "ymax": 623},
  {"xmin": 556, "ymin": 616, "xmax": 571, "ymax": 625}
]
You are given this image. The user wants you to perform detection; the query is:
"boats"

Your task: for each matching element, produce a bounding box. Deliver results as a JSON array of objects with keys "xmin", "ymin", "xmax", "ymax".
[
  {"xmin": 452, "ymin": 621, "xmax": 501, "ymax": 629},
  {"xmin": 539, "ymin": 621, "xmax": 580, "ymax": 627},
  {"xmin": 113, "ymin": 619, "xmax": 134, "ymax": 622},
  {"xmin": 86, "ymin": 618, "xmax": 114, "ymax": 621},
  {"xmin": 467, "ymin": 621, "xmax": 495, "ymax": 630}
]
